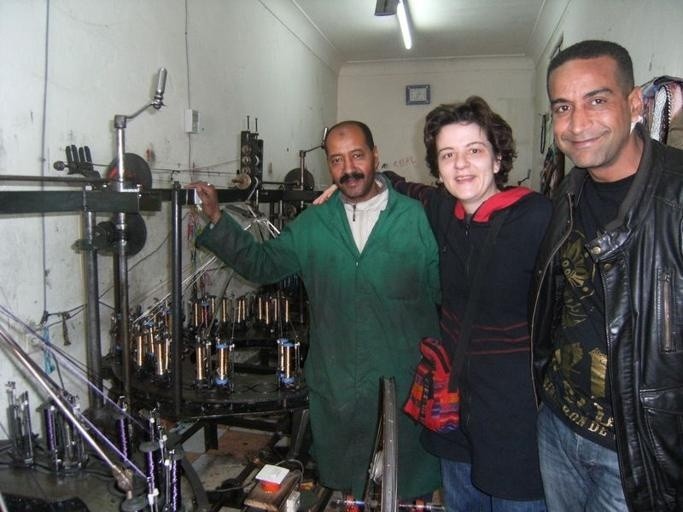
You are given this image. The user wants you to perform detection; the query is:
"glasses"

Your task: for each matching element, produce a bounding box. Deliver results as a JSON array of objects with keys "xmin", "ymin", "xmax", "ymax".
[
  {"xmin": 292, "ymin": 127, "xmax": 329, "ymax": 191},
  {"xmin": 397, "ymin": 0, "xmax": 414, "ymax": 50},
  {"xmin": 113, "ymin": 67, "xmax": 168, "ymax": 192}
]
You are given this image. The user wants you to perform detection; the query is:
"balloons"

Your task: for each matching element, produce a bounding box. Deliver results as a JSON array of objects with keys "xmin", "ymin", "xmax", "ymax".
[{"xmin": 405, "ymin": 84, "xmax": 431, "ymax": 104}]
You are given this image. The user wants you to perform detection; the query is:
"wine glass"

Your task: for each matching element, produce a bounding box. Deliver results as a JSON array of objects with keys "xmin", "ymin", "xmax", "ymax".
[{"xmin": 207, "ymin": 183, "xmax": 210, "ymax": 186}]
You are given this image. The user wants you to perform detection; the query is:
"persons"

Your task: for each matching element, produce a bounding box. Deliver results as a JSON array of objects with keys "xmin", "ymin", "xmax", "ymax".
[
  {"xmin": 312, "ymin": 93, "xmax": 548, "ymax": 509},
  {"xmin": 522, "ymin": 36, "xmax": 682, "ymax": 512},
  {"xmin": 182, "ymin": 117, "xmax": 449, "ymax": 510}
]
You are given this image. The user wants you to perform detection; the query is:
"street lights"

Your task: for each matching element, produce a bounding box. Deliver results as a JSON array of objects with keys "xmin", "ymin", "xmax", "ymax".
[{"xmin": 400, "ymin": 336, "xmax": 462, "ymax": 436}]
